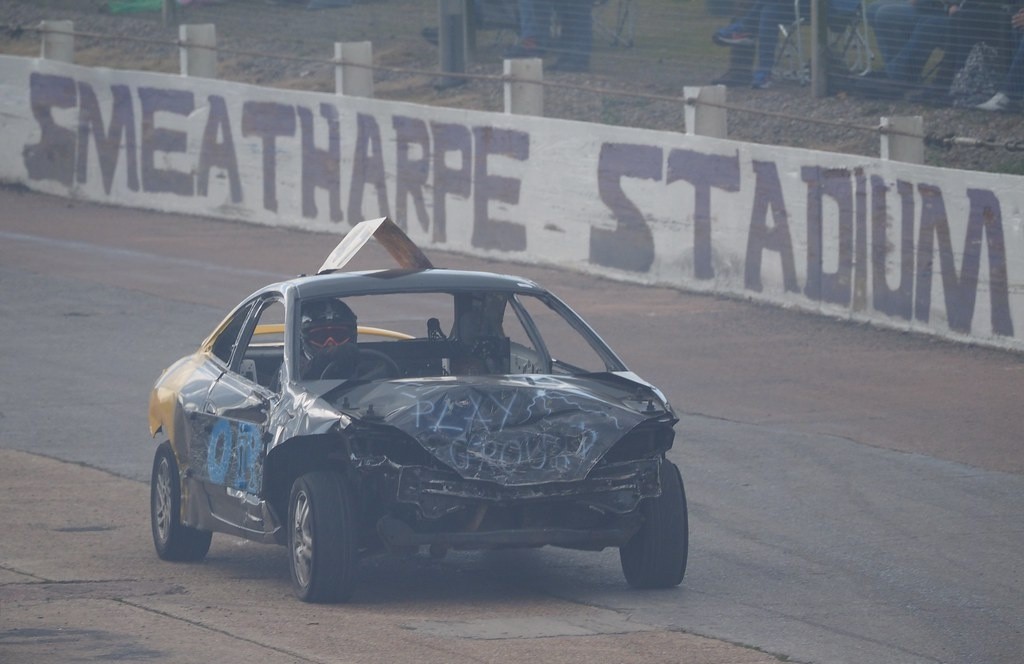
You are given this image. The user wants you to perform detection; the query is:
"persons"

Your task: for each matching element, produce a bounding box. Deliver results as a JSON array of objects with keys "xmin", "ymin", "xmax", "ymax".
[
  {"xmin": 424, "ymin": 0, "xmax": 593, "ymax": 70},
  {"xmin": 709, "ymin": 0, "xmax": 1024, "ymax": 113},
  {"xmin": 273, "ymin": 298, "xmax": 357, "ymax": 393}
]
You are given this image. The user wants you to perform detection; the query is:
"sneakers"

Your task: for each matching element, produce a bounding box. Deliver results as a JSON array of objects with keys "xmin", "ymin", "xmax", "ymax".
[
  {"xmin": 712, "ymin": 22, "xmax": 756, "ymax": 48},
  {"xmin": 750, "ymin": 63, "xmax": 774, "ymax": 88}
]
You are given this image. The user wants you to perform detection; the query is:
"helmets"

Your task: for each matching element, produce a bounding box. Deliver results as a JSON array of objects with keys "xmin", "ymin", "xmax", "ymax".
[{"xmin": 300, "ymin": 298, "xmax": 358, "ymax": 360}]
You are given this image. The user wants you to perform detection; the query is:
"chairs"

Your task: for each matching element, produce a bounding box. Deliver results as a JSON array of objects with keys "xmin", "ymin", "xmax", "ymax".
[{"xmin": 715, "ymin": 0, "xmax": 874, "ymax": 87}]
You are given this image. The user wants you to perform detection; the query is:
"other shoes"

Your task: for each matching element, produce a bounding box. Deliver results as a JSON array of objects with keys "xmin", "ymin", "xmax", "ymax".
[
  {"xmin": 422, "ymin": 26, "xmax": 439, "ymax": 46},
  {"xmin": 505, "ymin": 35, "xmax": 555, "ymax": 55},
  {"xmin": 974, "ymin": 91, "xmax": 1020, "ymax": 112},
  {"xmin": 712, "ymin": 68, "xmax": 751, "ymax": 86},
  {"xmin": 902, "ymin": 83, "xmax": 951, "ymax": 102},
  {"xmin": 544, "ymin": 53, "xmax": 591, "ymax": 72}
]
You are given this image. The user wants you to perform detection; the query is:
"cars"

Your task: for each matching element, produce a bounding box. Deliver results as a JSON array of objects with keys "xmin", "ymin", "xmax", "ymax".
[{"xmin": 147, "ymin": 264, "xmax": 691, "ymax": 605}]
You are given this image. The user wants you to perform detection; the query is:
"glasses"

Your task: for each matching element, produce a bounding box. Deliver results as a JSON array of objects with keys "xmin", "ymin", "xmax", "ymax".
[{"xmin": 307, "ymin": 328, "xmax": 353, "ymax": 347}]
